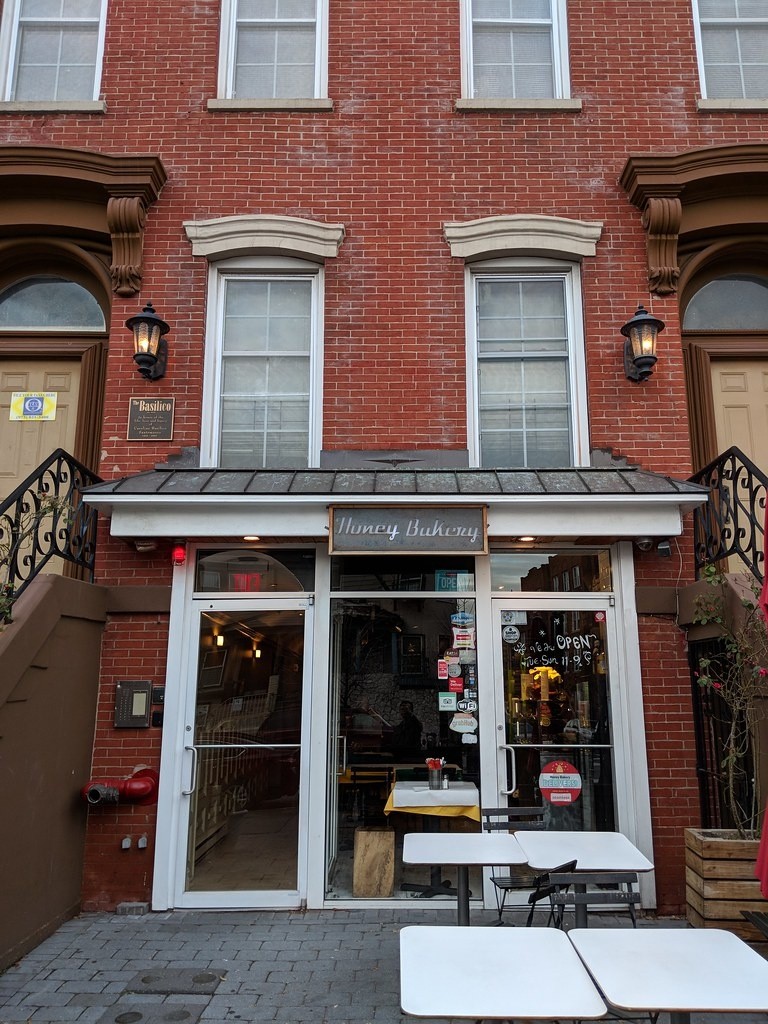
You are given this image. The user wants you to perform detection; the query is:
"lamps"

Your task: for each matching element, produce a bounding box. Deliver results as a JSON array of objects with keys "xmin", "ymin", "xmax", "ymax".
[
  {"xmin": 213, "ymin": 635, "xmax": 224, "ymax": 646},
  {"xmin": 253, "ymin": 650, "xmax": 261, "ymax": 658},
  {"xmin": 124, "ymin": 302, "xmax": 172, "ymax": 384},
  {"xmin": 619, "ymin": 303, "xmax": 665, "ymax": 384}
]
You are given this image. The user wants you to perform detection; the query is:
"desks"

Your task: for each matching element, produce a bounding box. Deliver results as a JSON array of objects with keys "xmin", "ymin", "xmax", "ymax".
[
  {"xmin": 402, "ymin": 833, "xmax": 528, "ymax": 927},
  {"xmin": 514, "ymin": 831, "xmax": 654, "ymax": 929},
  {"xmin": 339, "ymin": 764, "xmax": 463, "ymax": 836},
  {"xmin": 384, "ymin": 781, "xmax": 480, "ymax": 898},
  {"xmin": 513, "ymin": 742, "xmax": 603, "ymax": 831},
  {"xmin": 566, "ymin": 928, "xmax": 768, "ymax": 1024},
  {"xmin": 399, "ymin": 925, "xmax": 608, "ymax": 1024}
]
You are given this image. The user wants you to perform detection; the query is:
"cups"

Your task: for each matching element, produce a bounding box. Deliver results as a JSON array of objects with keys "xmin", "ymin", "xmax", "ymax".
[{"xmin": 427, "ymin": 770, "xmax": 441, "ymax": 790}]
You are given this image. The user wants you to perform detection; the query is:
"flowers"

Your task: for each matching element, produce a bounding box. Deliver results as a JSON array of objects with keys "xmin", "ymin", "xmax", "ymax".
[
  {"xmin": 691, "ymin": 555, "xmax": 768, "ymax": 839},
  {"xmin": 426, "ymin": 756, "xmax": 446, "ymax": 770}
]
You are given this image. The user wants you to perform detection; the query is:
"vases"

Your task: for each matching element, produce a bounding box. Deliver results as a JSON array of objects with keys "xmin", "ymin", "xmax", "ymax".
[{"xmin": 684, "ymin": 828, "xmax": 768, "ymax": 961}]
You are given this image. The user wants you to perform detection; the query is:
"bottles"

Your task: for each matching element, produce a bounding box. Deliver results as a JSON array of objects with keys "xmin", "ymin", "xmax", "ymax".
[
  {"xmin": 593, "ymin": 640, "xmax": 605, "ymax": 674},
  {"xmin": 442, "ymin": 774, "xmax": 449, "ymax": 789}
]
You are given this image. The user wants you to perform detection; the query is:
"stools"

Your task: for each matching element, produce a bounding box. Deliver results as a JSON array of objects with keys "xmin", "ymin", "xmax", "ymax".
[{"xmin": 352, "ymin": 826, "xmax": 396, "ymax": 897}]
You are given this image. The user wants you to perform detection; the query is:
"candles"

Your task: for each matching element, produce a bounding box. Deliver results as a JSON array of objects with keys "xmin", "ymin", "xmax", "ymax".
[
  {"xmin": 517, "ymin": 721, "xmax": 519, "ymax": 736},
  {"xmin": 516, "ymin": 703, "xmax": 518, "ymax": 713}
]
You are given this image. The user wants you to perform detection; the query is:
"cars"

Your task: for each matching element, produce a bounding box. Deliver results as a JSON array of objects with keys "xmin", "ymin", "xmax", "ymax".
[{"xmin": 196, "ymin": 731, "xmax": 300, "ymax": 816}]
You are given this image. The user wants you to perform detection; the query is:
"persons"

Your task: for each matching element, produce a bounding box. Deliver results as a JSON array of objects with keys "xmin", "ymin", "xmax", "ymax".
[{"xmin": 381, "ymin": 702, "xmax": 422, "ymax": 748}]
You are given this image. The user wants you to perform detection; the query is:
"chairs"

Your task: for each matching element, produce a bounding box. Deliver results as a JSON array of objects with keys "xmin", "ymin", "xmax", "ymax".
[
  {"xmin": 549, "ymin": 872, "xmax": 641, "ymax": 930},
  {"xmin": 408, "ymin": 767, "xmax": 456, "ymax": 832},
  {"xmin": 525, "ymin": 858, "xmax": 578, "ymax": 927},
  {"xmin": 481, "ymin": 807, "xmax": 557, "ymax": 927},
  {"xmin": 350, "ymin": 766, "xmax": 394, "ymax": 830}
]
